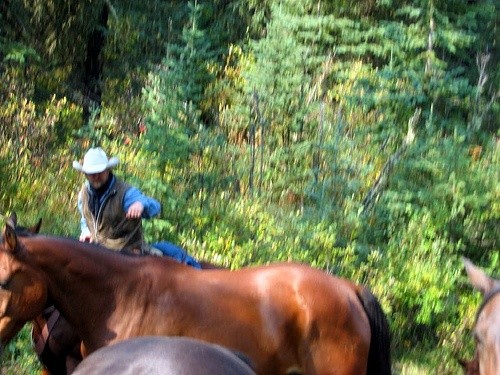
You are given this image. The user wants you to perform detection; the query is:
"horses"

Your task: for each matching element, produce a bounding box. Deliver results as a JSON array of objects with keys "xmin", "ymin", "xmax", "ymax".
[
  {"xmin": 455, "ymin": 255, "xmax": 500, "ymax": 375},
  {"xmin": 0, "ymin": 211, "xmax": 393, "ymax": 375}
]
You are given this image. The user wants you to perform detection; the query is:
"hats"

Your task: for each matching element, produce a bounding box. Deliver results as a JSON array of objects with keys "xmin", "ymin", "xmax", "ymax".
[{"xmin": 72, "ymin": 148, "xmax": 120, "ymax": 174}]
